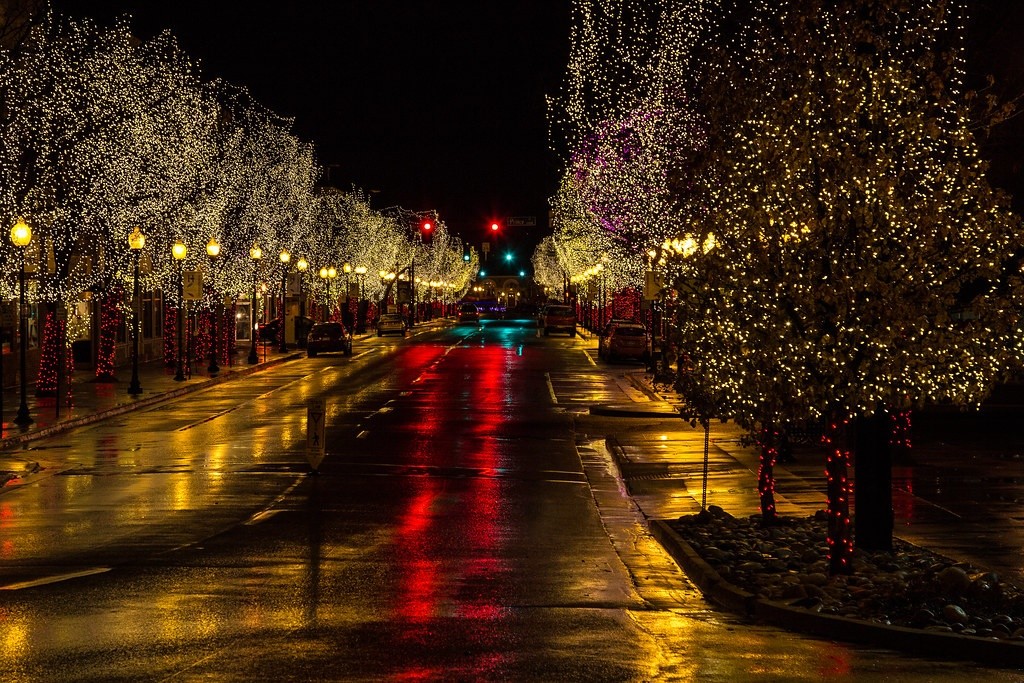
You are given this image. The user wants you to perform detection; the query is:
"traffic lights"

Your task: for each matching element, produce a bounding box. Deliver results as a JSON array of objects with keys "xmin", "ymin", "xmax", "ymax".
[
  {"xmin": 489, "ymin": 217, "xmax": 501, "ymax": 240},
  {"xmin": 463, "ymin": 242, "xmax": 472, "ymax": 262},
  {"xmin": 421, "ymin": 221, "xmax": 433, "ymax": 243}
]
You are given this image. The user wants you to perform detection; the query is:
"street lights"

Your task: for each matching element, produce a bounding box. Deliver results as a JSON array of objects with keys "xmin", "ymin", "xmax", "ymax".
[
  {"xmin": 296, "ymin": 259, "xmax": 309, "ymax": 349},
  {"xmin": 9, "ymin": 216, "xmax": 34, "ymax": 426},
  {"xmin": 248, "ymin": 245, "xmax": 262, "ymax": 364},
  {"xmin": 343, "ymin": 263, "xmax": 456, "ymax": 337},
  {"xmin": 170, "ymin": 239, "xmax": 187, "ymax": 382},
  {"xmin": 320, "ymin": 266, "xmax": 337, "ymax": 322},
  {"xmin": 126, "ymin": 226, "xmax": 146, "ymax": 394},
  {"xmin": 280, "ymin": 248, "xmax": 291, "ymax": 354},
  {"xmin": 205, "ymin": 238, "xmax": 222, "ymax": 373}
]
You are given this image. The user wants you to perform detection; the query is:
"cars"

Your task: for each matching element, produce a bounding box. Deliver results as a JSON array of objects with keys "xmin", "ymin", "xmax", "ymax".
[
  {"xmin": 538, "ymin": 305, "xmax": 576, "ymax": 337},
  {"xmin": 375, "ymin": 313, "xmax": 408, "ymax": 336},
  {"xmin": 457, "ymin": 303, "xmax": 480, "ymax": 324},
  {"xmin": 597, "ymin": 321, "xmax": 650, "ymax": 364}
]
